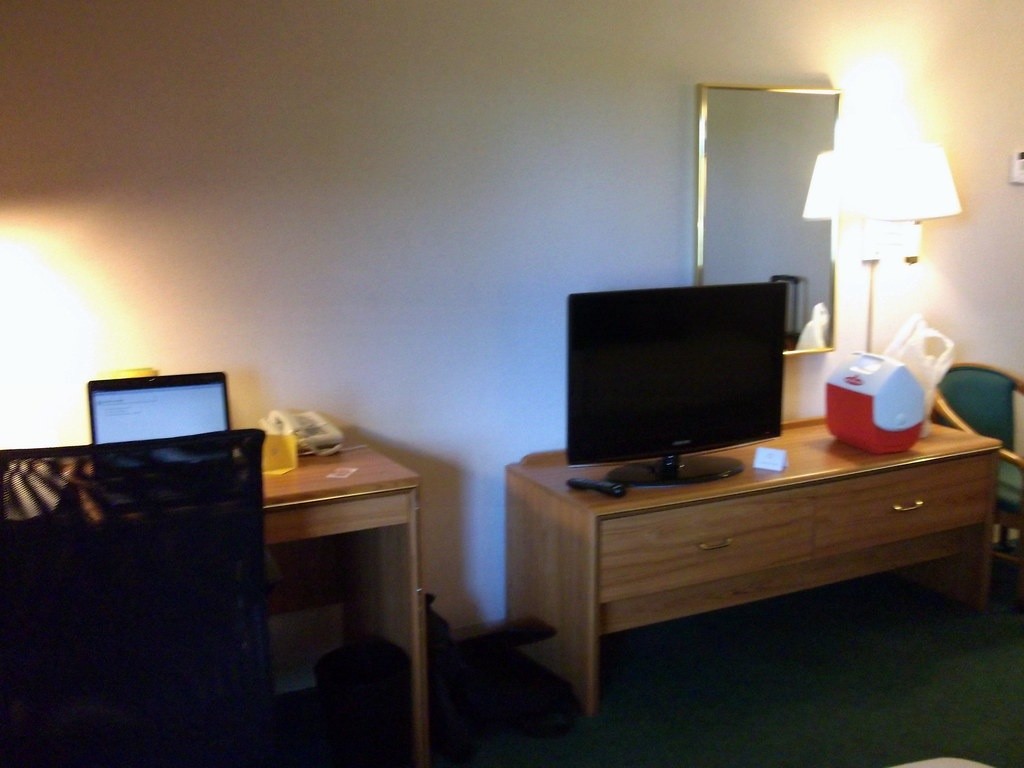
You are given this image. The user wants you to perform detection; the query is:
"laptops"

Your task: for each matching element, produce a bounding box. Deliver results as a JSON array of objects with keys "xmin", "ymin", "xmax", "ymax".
[{"xmin": 89, "ymin": 371, "xmax": 229, "ymax": 444}]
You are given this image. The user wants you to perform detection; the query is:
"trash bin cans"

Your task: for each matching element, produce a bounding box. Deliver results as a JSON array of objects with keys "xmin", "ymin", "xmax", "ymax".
[{"xmin": 313, "ymin": 638, "xmax": 413, "ymax": 768}]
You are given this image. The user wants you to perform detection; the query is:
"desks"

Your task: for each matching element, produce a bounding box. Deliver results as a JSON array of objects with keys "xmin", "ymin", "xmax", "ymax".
[{"xmin": 262, "ymin": 435, "xmax": 432, "ymax": 767}]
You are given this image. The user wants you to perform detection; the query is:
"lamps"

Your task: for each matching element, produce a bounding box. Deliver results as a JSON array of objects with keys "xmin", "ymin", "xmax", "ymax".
[{"xmin": 803, "ymin": 138, "xmax": 964, "ymax": 354}]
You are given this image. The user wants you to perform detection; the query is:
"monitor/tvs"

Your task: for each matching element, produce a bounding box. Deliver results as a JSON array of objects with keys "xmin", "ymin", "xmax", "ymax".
[{"xmin": 567, "ymin": 282, "xmax": 787, "ymax": 485}]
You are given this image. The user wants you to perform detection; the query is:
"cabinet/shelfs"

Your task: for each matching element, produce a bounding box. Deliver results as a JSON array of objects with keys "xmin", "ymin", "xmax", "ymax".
[{"xmin": 505, "ymin": 416, "xmax": 1003, "ymax": 717}]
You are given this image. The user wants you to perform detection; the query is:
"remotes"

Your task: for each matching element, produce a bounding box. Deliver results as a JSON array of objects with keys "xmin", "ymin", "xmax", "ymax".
[{"xmin": 567, "ymin": 478, "xmax": 625, "ymax": 499}]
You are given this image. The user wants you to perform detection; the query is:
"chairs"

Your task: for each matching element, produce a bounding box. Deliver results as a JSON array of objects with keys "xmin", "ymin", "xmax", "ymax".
[
  {"xmin": 0, "ymin": 428, "xmax": 329, "ymax": 768},
  {"xmin": 932, "ymin": 363, "xmax": 1024, "ymax": 611}
]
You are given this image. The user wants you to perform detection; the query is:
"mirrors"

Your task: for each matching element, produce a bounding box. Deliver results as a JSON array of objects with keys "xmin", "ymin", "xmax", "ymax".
[{"xmin": 692, "ymin": 84, "xmax": 843, "ymax": 358}]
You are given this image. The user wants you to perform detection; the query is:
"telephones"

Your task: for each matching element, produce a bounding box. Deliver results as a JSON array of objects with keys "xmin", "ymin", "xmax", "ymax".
[{"xmin": 257, "ymin": 410, "xmax": 345, "ymax": 453}]
